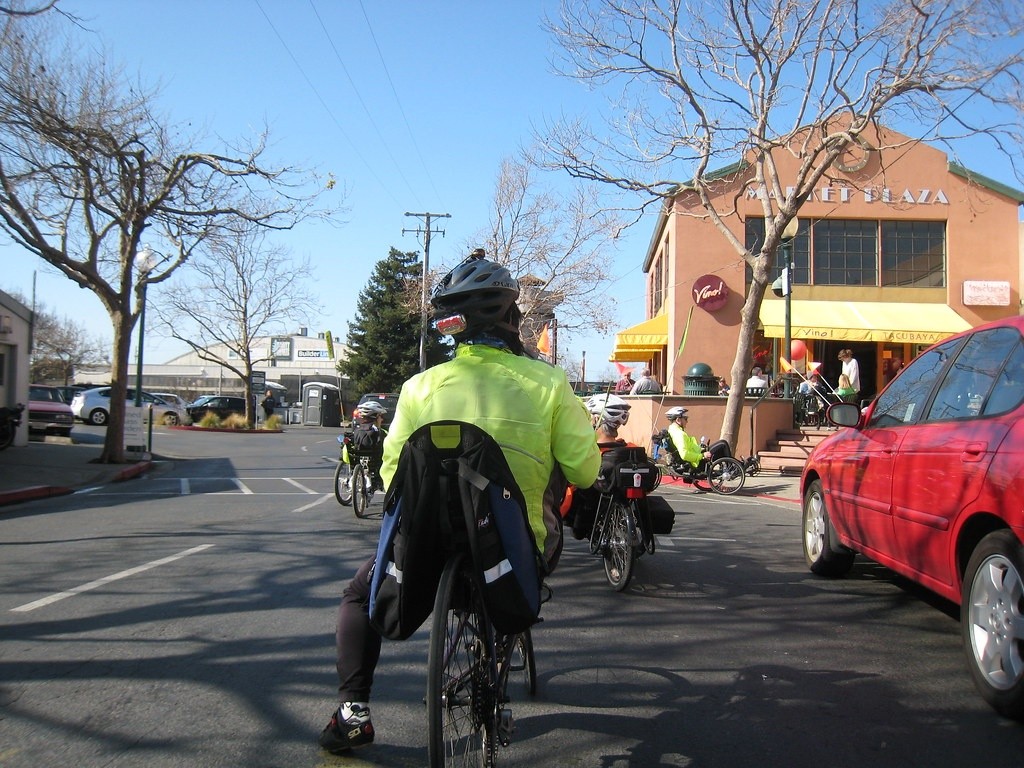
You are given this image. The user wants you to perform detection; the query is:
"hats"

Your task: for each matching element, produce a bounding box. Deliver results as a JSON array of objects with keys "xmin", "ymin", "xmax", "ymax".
[{"xmin": 807, "ymin": 371, "xmax": 814, "ymax": 379}]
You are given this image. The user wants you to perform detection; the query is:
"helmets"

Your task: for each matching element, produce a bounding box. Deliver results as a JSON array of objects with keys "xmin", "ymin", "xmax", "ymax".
[
  {"xmin": 431, "ymin": 260, "xmax": 520, "ymax": 321},
  {"xmin": 357, "ymin": 401, "xmax": 387, "ymax": 418},
  {"xmin": 586, "ymin": 392, "xmax": 631, "ymax": 425},
  {"xmin": 665, "ymin": 407, "xmax": 689, "ymax": 422}
]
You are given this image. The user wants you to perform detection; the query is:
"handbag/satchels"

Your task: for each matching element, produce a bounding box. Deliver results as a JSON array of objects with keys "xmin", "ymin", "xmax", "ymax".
[
  {"xmin": 370, "ymin": 416, "xmax": 546, "ymax": 644},
  {"xmin": 631, "ymin": 495, "xmax": 674, "ymax": 536}
]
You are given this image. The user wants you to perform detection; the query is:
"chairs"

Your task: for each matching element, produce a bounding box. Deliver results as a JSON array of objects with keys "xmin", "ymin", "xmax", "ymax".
[
  {"xmin": 953, "ymin": 356, "xmax": 1015, "ymax": 413},
  {"xmin": 574, "ymin": 386, "xmax": 859, "ymax": 430}
]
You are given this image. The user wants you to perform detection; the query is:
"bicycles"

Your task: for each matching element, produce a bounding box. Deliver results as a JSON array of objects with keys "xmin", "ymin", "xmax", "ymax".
[
  {"xmin": 587, "ymin": 445, "xmax": 658, "ymax": 593},
  {"xmin": 366, "ymin": 421, "xmax": 536, "ymax": 768}
]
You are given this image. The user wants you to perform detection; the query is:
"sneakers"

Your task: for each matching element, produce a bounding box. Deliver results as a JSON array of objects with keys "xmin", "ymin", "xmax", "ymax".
[{"xmin": 318, "ymin": 712, "xmax": 374, "ymax": 752}]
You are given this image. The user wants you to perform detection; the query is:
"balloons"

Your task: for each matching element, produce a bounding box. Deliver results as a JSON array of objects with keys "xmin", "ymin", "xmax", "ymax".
[{"xmin": 791, "ymin": 340, "xmax": 807, "ymax": 361}]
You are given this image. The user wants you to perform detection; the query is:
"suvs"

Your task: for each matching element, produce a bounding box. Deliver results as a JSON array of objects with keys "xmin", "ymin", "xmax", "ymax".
[{"xmin": 352, "ymin": 393, "xmax": 400, "ymax": 432}]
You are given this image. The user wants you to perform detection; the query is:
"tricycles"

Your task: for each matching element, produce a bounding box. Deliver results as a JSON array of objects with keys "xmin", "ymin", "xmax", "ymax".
[
  {"xmin": 646, "ymin": 430, "xmax": 763, "ymax": 495},
  {"xmin": 334, "ymin": 432, "xmax": 384, "ymax": 518}
]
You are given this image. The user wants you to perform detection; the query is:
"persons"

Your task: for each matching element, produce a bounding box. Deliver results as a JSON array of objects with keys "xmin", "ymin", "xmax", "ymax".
[
  {"xmin": 320, "ymin": 250, "xmax": 601, "ymax": 753},
  {"xmin": 747, "ymin": 367, "xmax": 768, "ymax": 394},
  {"xmin": 261, "ymin": 391, "xmax": 275, "ymax": 419},
  {"xmin": 839, "ymin": 350, "xmax": 860, "ymax": 393},
  {"xmin": 665, "ymin": 407, "xmax": 734, "ymax": 471},
  {"xmin": 355, "ymin": 402, "xmax": 387, "ymax": 475},
  {"xmin": 838, "ymin": 373, "xmax": 854, "ymax": 398},
  {"xmin": 630, "ymin": 368, "xmax": 661, "ymax": 395},
  {"xmin": 616, "ymin": 371, "xmax": 635, "ymax": 395},
  {"xmin": 558, "ymin": 393, "xmax": 637, "ymax": 520},
  {"xmin": 800, "ymin": 377, "xmax": 824, "ymax": 393}
]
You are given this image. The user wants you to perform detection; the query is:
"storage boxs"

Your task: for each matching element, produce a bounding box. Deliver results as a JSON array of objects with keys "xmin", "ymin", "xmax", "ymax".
[
  {"xmin": 601, "ymin": 447, "xmax": 656, "ymax": 489},
  {"xmin": 640, "ymin": 496, "xmax": 675, "ymax": 534}
]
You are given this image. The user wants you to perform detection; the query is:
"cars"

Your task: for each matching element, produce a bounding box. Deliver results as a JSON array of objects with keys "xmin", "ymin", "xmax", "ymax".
[
  {"xmin": 134, "ymin": 391, "xmax": 188, "ymax": 409},
  {"xmin": 186, "ymin": 394, "xmax": 245, "ymax": 423},
  {"xmin": 800, "ymin": 315, "xmax": 1023, "ymax": 713},
  {"xmin": 71, "ymin": 385, "xmax": 192, "ymax": 427},
  {"xmin": 28, "ymin": 384, "xmax": 74, "ymax": 436},
  {"xmin": 56, "ymin": 386, "xmax": 89, "ymax": 404}
]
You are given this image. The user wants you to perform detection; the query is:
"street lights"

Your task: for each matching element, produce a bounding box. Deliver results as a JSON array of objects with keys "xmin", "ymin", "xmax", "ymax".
[
  {"xmin": 127, "ymin": 244, "xmax": 157, "ymax": 453},
  {"xmin": 774, "ymin": 212, "xmax": 798, "ymax": 396}
]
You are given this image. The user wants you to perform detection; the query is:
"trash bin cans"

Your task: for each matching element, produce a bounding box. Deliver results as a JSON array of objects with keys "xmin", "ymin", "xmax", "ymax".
[
  {"xmin": 681, "ymin": 363, "xmax": 722, "ymax": 396},
  {"xmin": 587, "ymin": 386, "xmax": 605, "ymax": 396},
  {"xmin": 575, "ymin": 391, "xmax": 590, "ymax": 397},
  {"xmin": 745, "ymin": 387, "xmax": 767, "ymax": 394}
]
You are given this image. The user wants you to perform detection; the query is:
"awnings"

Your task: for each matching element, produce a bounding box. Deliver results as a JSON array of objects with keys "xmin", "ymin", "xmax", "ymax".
[
  {"xmin": 760, "ymin": 300, "xmax": 973, "ymax": 344},
  {"xmin": 609, "ymin": 313, "xmax": 668, "ymax": 362}
]
